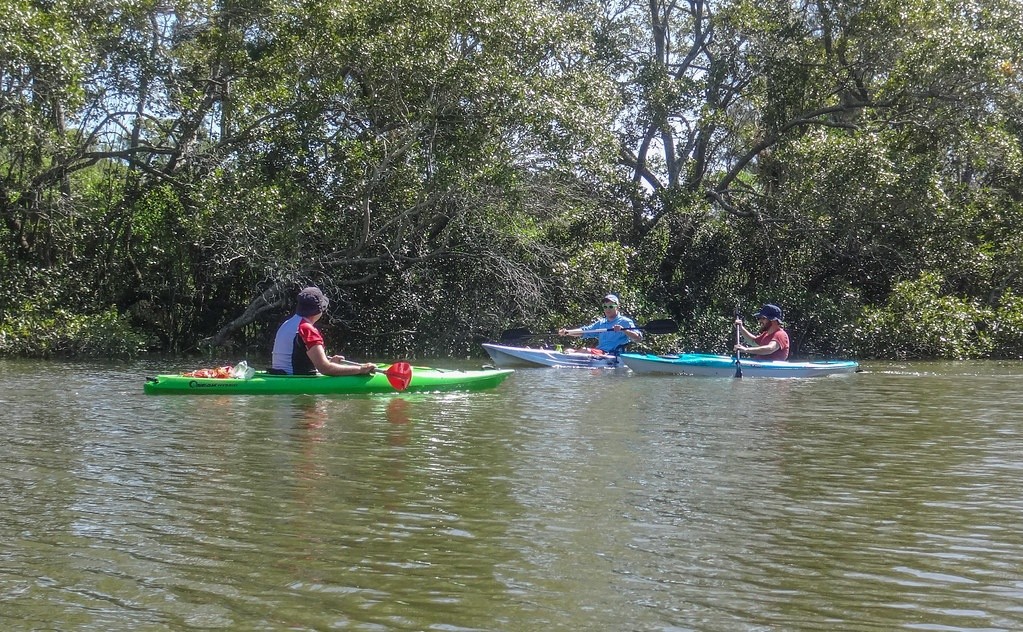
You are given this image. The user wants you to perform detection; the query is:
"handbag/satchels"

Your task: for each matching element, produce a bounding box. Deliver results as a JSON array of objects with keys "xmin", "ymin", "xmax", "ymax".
[{"xmin": 232, "ymin": 360, "xmax": 255, "ymax": 379}]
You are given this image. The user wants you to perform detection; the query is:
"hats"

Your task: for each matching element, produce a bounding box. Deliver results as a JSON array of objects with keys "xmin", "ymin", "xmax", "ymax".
[
  {"xmin": 295, "ymin": 287, "xmax": 330, "ymax": 317},
  {"xmin": 602, "ymin": 295, "xmax": 619, "ymax": 304},
  {"xmin": 754, "ymin": 304, "xmax": 784, "ymax": 325}
]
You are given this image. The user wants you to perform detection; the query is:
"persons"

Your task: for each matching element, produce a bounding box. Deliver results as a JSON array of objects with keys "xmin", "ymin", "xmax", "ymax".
[
  {"xmin": 734, "ymin": 304, "xmax": 789, "ymax": 361},
  {"xmin": 271, "ymin": 287, "xmax": 377, "ymax": 375},
  {"xmin": 559, "ymin": 295, "xmax": 642, "ymax": 352}
]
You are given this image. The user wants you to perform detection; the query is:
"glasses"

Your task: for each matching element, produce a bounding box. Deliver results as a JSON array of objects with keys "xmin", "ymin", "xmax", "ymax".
[
  {"xmin": 602, "ymin": 302, "xmax": 617, "ymax": 309},
  {"xmin": 760, "ymin": 317, "xmax": 767, "ymax": 320}
]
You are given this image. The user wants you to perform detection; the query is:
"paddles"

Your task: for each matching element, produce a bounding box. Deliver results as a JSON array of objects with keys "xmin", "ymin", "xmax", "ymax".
[
  {"xmin": 498, "ymin": 318, "xmax": 681, "ymax": 345},
  {"xmin": 325, "ymin": 354, "xmax": 414, "ymax": 391},
  {"xmin": 734, "ymin": 306, "xmax": 743, "ymax": 378}
]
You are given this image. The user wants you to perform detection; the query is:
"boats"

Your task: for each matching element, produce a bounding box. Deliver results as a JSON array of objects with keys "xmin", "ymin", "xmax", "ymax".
[
  {"xmin": 620, "ymin": 353, "xmax": 859, "ymax": 378},
  {"xmin": 143, "ymin": 363, "xmax": 515, "ymax": 395},
  {"xmin": 482, "ymin": 343, "xmax": 621, "ymax": 368}
]
